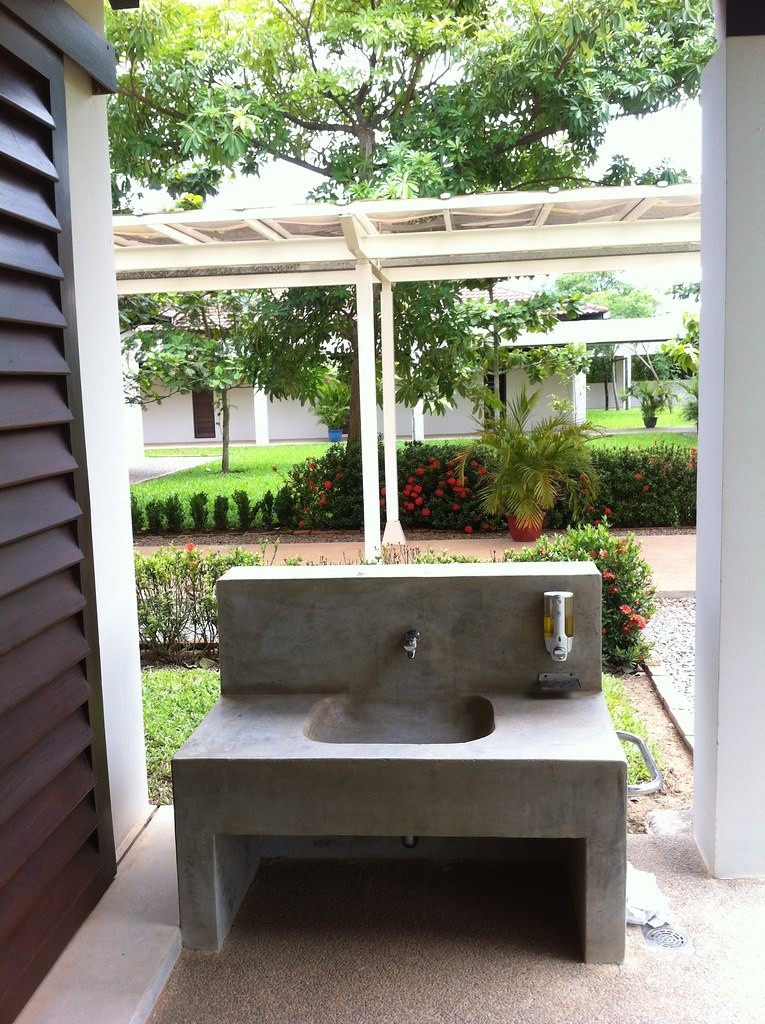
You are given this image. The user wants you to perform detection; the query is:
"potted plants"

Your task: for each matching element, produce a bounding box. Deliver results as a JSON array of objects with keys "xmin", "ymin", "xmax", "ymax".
[
  {"xmin": 618, "ymin": 380, "xmax": 684, "ymax": 429},
  {"xmin": 451, "ymin": 378, "xmax": 602, "ymax": 542},
  {"xmin": 309, "ymin": 378, "xmax": 352, "ymax": 443}
]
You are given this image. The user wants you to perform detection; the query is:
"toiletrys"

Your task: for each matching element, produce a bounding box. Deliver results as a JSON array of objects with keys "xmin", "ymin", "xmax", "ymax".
[{"xmin": 542, "ymin": 590, "xmax": 574, "ymax": 662}]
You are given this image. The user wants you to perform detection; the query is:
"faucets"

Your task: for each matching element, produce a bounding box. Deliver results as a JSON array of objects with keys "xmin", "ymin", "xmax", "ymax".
[{"xmin": 403, "ymin": 628, "xmax": 420, "ymax": 660}]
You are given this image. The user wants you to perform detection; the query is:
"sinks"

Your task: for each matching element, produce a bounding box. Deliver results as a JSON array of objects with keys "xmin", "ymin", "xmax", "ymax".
[{"xmin": 301, "ymin": 692, "xmax": 495, "ymax": 745}]
